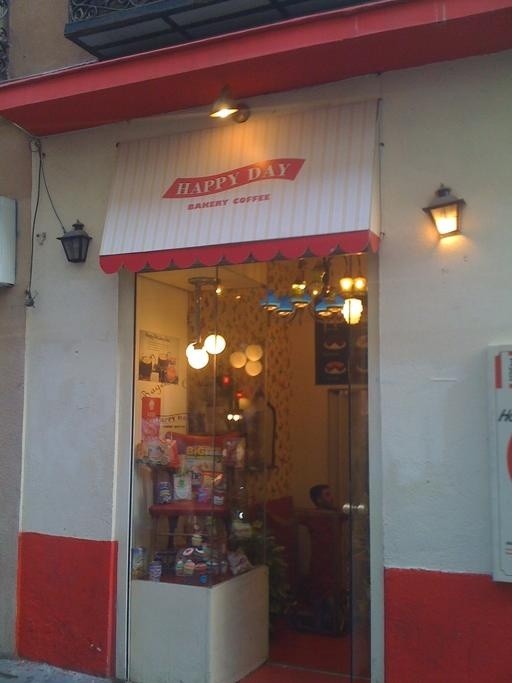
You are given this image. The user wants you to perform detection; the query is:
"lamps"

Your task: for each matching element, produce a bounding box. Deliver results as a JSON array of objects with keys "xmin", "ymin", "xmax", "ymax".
[
  {"xmin": 185, "ymin": 274, "xmax": 227, "ymax": 370},
  {"xmin": 209, "ymin": 83, "xmax": 252, "ymax": 124},
  {"xmin": 423, "ymin": 180, "xmax": 468, "ymax": 239},
  {"xmin": 56, "ymin": 218, "xmax": 93, "ymax": 264},
  {"xmin": 260, "ymin": 255, "xmax": 346, "ymax": 322}
]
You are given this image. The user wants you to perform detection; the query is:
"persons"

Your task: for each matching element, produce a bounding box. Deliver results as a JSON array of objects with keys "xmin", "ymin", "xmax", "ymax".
[{"xmin": 309, "ymin": 483, "xmax": 342, "ymax": 513}]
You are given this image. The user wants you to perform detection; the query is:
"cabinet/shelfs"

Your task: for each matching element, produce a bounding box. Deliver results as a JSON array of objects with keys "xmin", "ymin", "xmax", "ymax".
[{"xmin": 133, "ymin": 561, "xmax": 272, "ymax": 682}]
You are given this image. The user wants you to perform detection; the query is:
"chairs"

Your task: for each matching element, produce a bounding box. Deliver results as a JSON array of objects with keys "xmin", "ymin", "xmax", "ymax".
[
  {"xmin": 250, "ymin": 495, "xmax": 351, "ymax": 636},
  {"xmin": 150, "ymin": 431, "xmax": 243, "ymax": 548}
]
